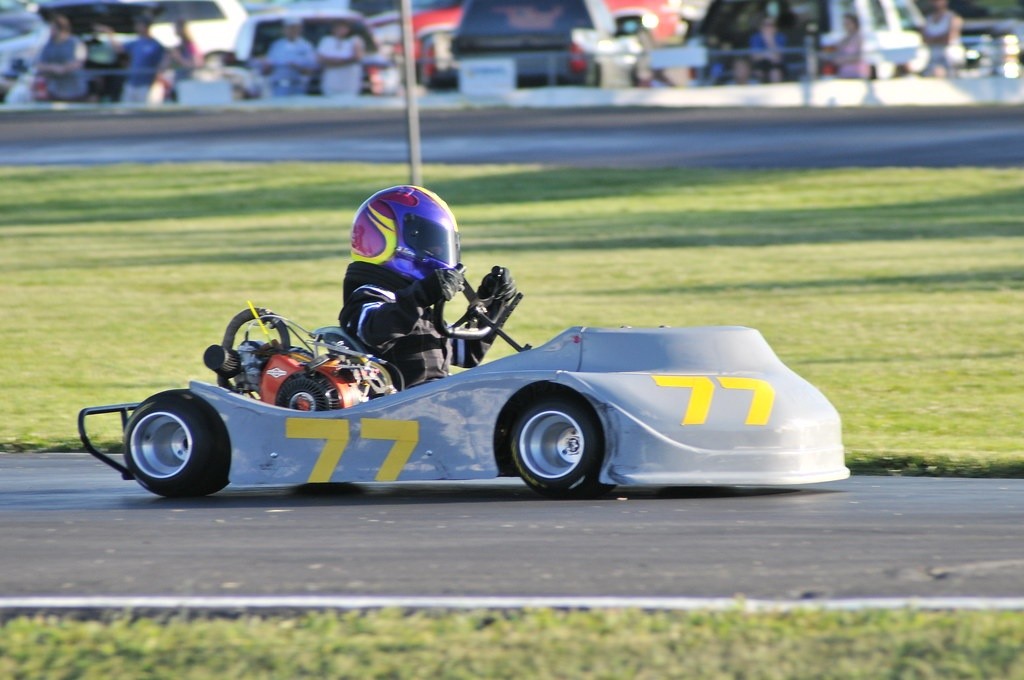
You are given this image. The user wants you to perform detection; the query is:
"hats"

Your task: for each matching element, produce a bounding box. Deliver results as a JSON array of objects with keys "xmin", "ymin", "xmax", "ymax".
[{"xmin": 285, "ymin": 15, "xmax": 301, "ymax": 26}]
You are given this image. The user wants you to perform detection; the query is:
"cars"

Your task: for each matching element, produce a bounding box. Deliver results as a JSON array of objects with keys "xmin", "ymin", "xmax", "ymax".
[{"xmin": 1, "ymin": 0, "xmax": 1024, "ymax": 115}]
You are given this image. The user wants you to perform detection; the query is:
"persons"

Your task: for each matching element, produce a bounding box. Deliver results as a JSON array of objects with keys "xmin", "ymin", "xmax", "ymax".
[
  {"xmin": 338, "ymin": 184, "xmax": 516, "ymax": 396},
  {"xmin": 35, "ymin": 16, "xmax": 366, "ymax": 99},
  {"xmin": 751, "ymin": 0, "xmax": 966, "ymax": 80}
]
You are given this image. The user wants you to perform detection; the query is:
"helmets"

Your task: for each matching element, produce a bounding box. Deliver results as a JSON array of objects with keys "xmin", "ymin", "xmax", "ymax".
[{"xmin": 350, "ymin": 184, "xmax": 461, "ymax": 279}]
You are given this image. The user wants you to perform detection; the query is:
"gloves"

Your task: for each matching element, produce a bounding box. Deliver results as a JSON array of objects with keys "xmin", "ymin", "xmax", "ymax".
[
  {"xmin": 417, "ymin": 267, "xmax": 465, "ymax": 303},
  {"xmin": 478, "ymin": 265, "xmax": 516, "ymax": 305}
]
